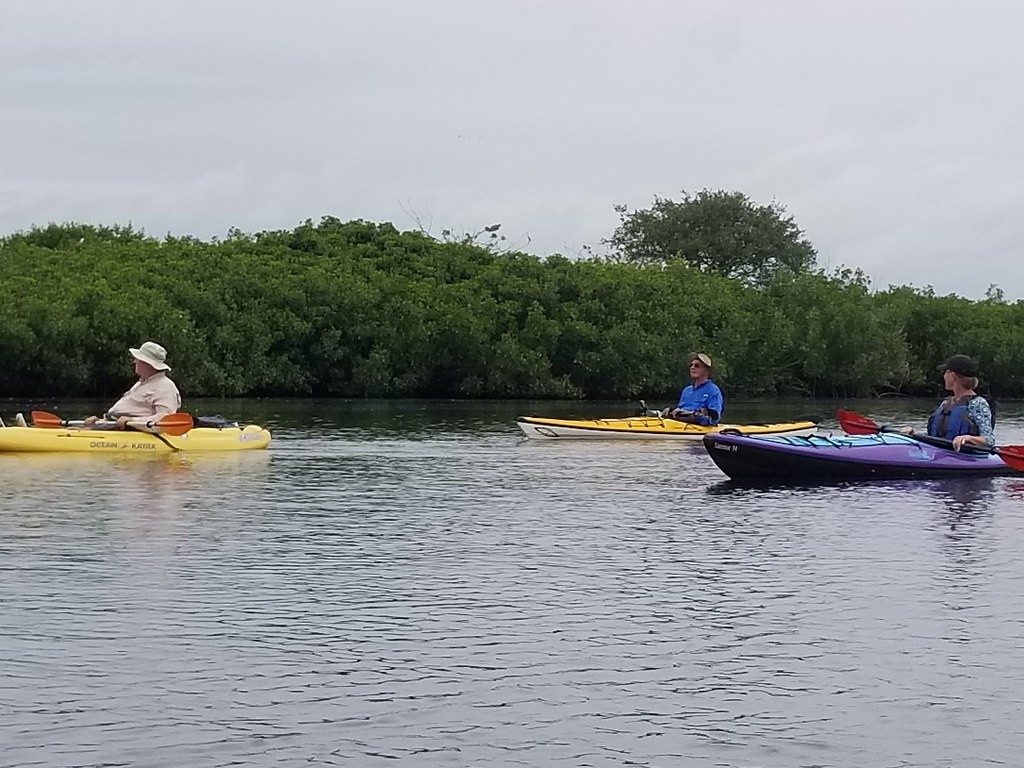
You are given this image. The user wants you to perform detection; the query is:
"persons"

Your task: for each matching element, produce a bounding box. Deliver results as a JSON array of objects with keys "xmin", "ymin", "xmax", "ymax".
[
  {"xmin": 662, "ymin": 353, "xmax": 723, "ymax": 427},
  {"xmin": 901, "ymin": 355, "xmax": 995, "ymax": 451},
  {"xmin": 0, "ymin": 341, "xmax": 181, "ymax": 430}
]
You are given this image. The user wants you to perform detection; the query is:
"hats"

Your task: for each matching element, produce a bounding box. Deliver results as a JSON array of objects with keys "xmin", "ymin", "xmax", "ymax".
[
  {"xmin": 688, "ymin": 352, "xmax": 711, "ymax": 367},
  {"xmin": 129, "ymin": 341, "xmax": 171, "ymax": 372},
  {"xmin": 936, "ymin": 355, "xmax": 977, "ymax": 377}
]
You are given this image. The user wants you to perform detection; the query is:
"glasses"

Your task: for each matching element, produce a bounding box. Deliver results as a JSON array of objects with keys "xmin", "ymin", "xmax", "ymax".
[
  {"xmin": 942, "ymin": 369, "xmax": 951, "ymax": 375},
  {"xmin": 689, "ymin": 363, "xmax": 703, "ymax": 368}
]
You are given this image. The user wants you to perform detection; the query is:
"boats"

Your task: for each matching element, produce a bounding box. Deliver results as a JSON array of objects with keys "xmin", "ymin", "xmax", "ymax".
[
  {"xmin": 702, "ymin": 428, "xmax": 1024, "ymax": 480},
  {"xmin": 517, "ymin": 414, "xmax": 821, "ymax": 440},
  {"xmin": 0, "ymin": 420, "xmax": 271, "ymax": 455}
]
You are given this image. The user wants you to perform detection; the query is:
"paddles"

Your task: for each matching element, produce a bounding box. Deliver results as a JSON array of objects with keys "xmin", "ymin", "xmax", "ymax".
[
  {"xmin": 833, "ymin": 408, "xmax": 1024, "ymax": 474},
  {"xmin": 29, "ymin": 409, "xmax": 194, "ymax": 436}
]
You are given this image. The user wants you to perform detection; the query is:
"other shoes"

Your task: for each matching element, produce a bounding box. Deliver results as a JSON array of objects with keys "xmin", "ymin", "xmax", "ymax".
[
  {"xmin": 0, "ymin": 417, "xmax": 8, "ymax": 427},
  {"xmin": 16, "ymin": 413, "xmax": 28, "ymax": 427}
]
